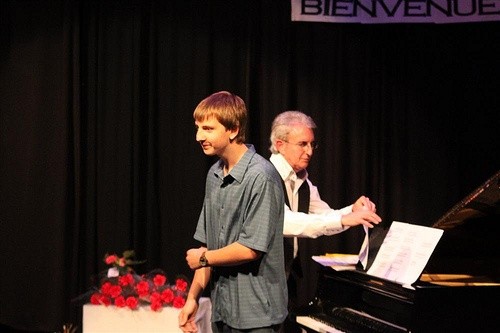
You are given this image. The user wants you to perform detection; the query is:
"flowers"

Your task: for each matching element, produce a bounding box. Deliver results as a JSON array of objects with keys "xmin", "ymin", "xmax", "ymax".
[{"xmin": 89, "ymin": 253, "xmax": 192, "ymax": 312}]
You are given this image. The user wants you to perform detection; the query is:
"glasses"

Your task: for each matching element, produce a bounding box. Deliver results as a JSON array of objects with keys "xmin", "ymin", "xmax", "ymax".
[{"xmin": 279, "ymin": 136, "xmax": 319, "ymax": 151}]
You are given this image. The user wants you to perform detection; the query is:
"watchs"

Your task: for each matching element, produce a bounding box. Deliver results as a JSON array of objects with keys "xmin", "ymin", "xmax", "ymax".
[{"xmin": 200, "ymin": 251, "xmax": 208, "ymax": 267}]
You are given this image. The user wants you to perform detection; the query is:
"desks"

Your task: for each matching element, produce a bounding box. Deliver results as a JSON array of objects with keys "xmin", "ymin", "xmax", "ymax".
[{"xmin": 82, "ymin": 297, "xmax": 212, "ymax": 333}]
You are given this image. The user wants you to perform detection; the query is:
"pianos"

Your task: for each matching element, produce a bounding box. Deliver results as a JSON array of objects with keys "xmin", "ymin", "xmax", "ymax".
[{"xmin": 286, "ymin": 173, "xmax": 500, "ymax": 333}]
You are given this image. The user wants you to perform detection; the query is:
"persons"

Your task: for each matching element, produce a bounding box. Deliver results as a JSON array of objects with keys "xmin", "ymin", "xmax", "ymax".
[
  {"xmin": 268, "ymin": 111, "xmax": 382, "ymax": 333},
  {"xmin": 179, "ymin": 91, "xmax": 289, "ymax": 333}
]
